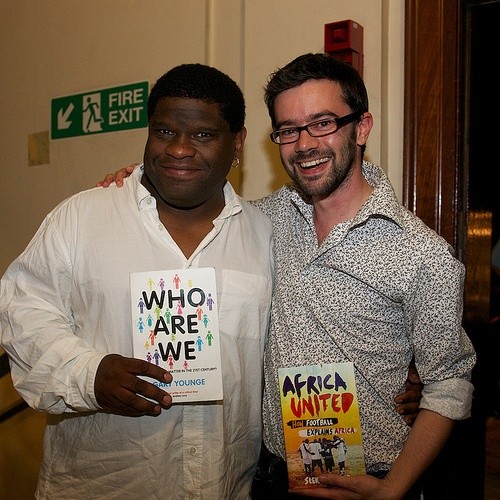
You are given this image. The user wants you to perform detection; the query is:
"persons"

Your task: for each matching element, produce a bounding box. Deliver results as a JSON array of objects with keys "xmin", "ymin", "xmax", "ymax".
[
  {"xmin": 298, "ymin": 435, "xmax": 348, "ymax": 479},
  {"xmin": 0, "ymin": 63, "xmax": 426, "ymax": 500},
  {"xmin": 94, "ymin": 53, "xmax": 476, "ymax": 500}
]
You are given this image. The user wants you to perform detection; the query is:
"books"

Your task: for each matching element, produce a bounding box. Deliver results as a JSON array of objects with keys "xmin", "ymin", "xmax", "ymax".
[
  {"xmin": 130, "ymin": 267, "xmax": 224, "ymax": 404},
  {"xmin": 277, "ymin": 363, "xmax": 367, "ymax": 490}
]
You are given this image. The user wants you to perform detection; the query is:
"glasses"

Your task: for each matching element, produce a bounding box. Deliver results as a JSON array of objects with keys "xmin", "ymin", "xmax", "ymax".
[{"xmin": 270, "ymin": 113, "xmax": 358, "ymax": 145}]
